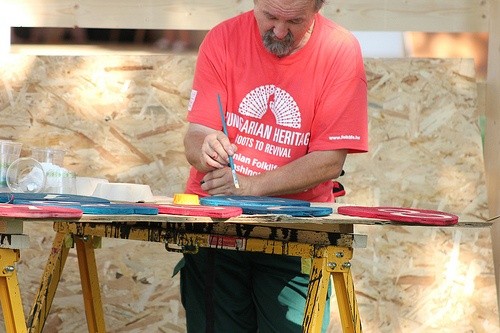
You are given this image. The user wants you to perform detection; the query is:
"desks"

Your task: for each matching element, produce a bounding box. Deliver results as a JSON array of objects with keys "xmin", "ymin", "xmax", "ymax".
[{"xmin": 0, "ymin": 203, "xmax": 493, "ymax": 333}]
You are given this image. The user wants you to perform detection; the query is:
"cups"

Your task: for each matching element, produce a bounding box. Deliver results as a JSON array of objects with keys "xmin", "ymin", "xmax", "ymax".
[
  {"xmin": 32, "ymin": 148, "xmax": 64, "ymax": 167},
  {"xmin": 0, "ymin": 140, "xmax": 21, "ymax": 186},
  {"xmin": 47, "ymin": 169, "xmax": 78, "ymax": 200},
  {"xmin": 6, "ymin": 157, "xmax": 47, "ymax": 194}
]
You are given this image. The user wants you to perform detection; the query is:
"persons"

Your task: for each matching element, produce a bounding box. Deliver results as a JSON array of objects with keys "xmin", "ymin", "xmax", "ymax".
[{"xmin": 171, "ymin": 0, "xmax": 369, "ymax": 333}]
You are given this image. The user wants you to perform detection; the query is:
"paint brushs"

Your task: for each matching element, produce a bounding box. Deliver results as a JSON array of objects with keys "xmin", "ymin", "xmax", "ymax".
[{"xmin": 216, "ymin": 94, "xmax": 239, "ymax": 190}]
